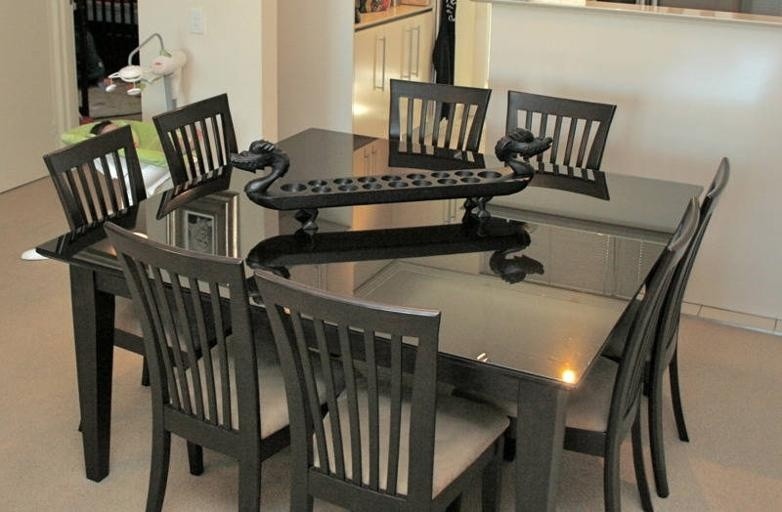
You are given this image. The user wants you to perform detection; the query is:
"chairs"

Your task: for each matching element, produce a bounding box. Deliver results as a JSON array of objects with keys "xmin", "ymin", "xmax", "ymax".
[
  {"xmin": 104, "ymin": 222, "xmax": 362, "ymax": 512},
  {"xmin": 600, "ymin": 157, "xmax": 731, "ymax": 496},
  {"xmin": 254, "ymin": 269, "xmax": 511, "ymax": 512},
  {"xmin": 388, "ymin": 78, "xmax": 492, "ymax": 153},
  {"xmin": 505, "ymin": 90, "xmax": 617, "ymax": 171},
  {"xmin": 451, "ymin": 196, "xmax": 700, "ymax": 512},
  {"xmin": 152, "ymin": 92, "xmax": 239, "ymax": 187},
  {"xmin": 42, "ymin": 125, "xmax": 231, "ymax": 475}
]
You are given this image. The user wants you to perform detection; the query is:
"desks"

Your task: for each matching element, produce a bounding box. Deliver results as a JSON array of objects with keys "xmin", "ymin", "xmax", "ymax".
[{"xmin": 35, "ymin": 127, "xmax": 703, "ymax": 512}]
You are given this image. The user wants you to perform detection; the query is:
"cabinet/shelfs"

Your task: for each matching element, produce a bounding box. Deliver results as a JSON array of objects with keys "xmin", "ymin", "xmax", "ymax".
[{"xmin": 352, "ymin": 11, "xmax": 436, "ymax": 138}]
